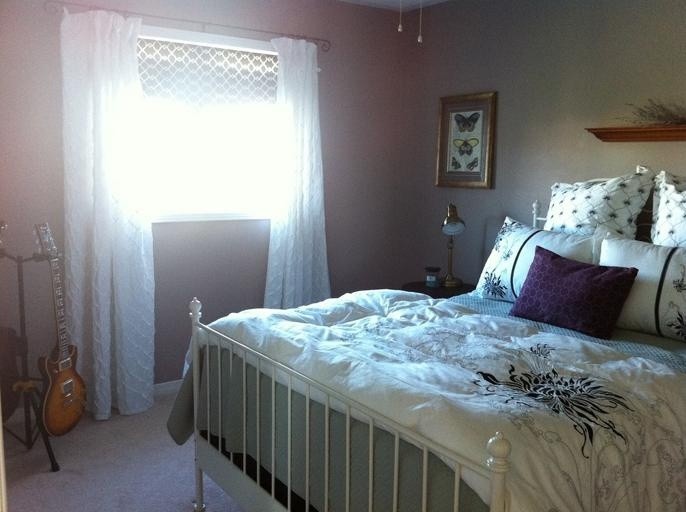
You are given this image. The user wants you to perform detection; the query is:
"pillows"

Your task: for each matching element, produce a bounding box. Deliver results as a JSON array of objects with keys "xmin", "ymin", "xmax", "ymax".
[
  {"xmin": 506, "ymin": 242, "xmax": 640, "ymax": 341},
  {"xmin": 543, "ymin": 162, "xmax": 652, "ymax": 241},
  {"xmin": 593, "ymin": 223, "xmax": 686, "ymax": 346},
  {"xmin": 468, "ymin": 212, "xmax": 594, "ymax": 305},
  {"xmin": 645, "ymin": 167, "xmax": 685, "ymax": 249}
]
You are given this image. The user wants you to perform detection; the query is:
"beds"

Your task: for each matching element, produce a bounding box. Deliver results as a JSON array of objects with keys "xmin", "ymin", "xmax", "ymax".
[{"xmin": 166, "ymin": 164, "xmax": 686, "ymax": 512}]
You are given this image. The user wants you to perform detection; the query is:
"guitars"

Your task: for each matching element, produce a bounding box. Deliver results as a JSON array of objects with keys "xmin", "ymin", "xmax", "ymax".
[{"xmin": 33, "ymin": 222, "xmax": 88, "ymax": 437}]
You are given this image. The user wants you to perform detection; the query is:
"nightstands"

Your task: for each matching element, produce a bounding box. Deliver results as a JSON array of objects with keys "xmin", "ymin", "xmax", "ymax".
[{"xmin": 400, "ymin": 277, "xmax": 475, "ymax": 302}]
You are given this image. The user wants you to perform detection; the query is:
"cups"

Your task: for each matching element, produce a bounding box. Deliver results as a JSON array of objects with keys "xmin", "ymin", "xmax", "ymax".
[{"xmin": 424, "ymin": 266, "xmax": 441, "ymax": 288}]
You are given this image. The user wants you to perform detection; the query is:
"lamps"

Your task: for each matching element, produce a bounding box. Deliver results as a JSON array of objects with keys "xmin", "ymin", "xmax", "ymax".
[{"xmin": 438, "ymin": 203, "xmax": 464, "ymax": 287}]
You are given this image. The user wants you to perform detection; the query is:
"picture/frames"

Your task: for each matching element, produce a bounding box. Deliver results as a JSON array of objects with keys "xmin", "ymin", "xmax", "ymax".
[{"xmin": 434, "ymin": 90, "xmax": 497, "ymax": 192}]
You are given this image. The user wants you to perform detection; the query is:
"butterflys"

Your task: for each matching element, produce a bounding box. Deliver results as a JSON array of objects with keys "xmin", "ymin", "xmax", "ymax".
[
  {"xmin": 454, "ymin": 112, "xmax": 480, "ymax": 133},
  {"xmin": 453, "ymin": 137, "xmax": 479, "ymax": 155},
  {"xmin": 451, "ymin": 157, "xmax": 461, "ymax": 169},
  {"xmin": 466, "ymin": 157, "xmax": 478, "ymax": 170}
]
